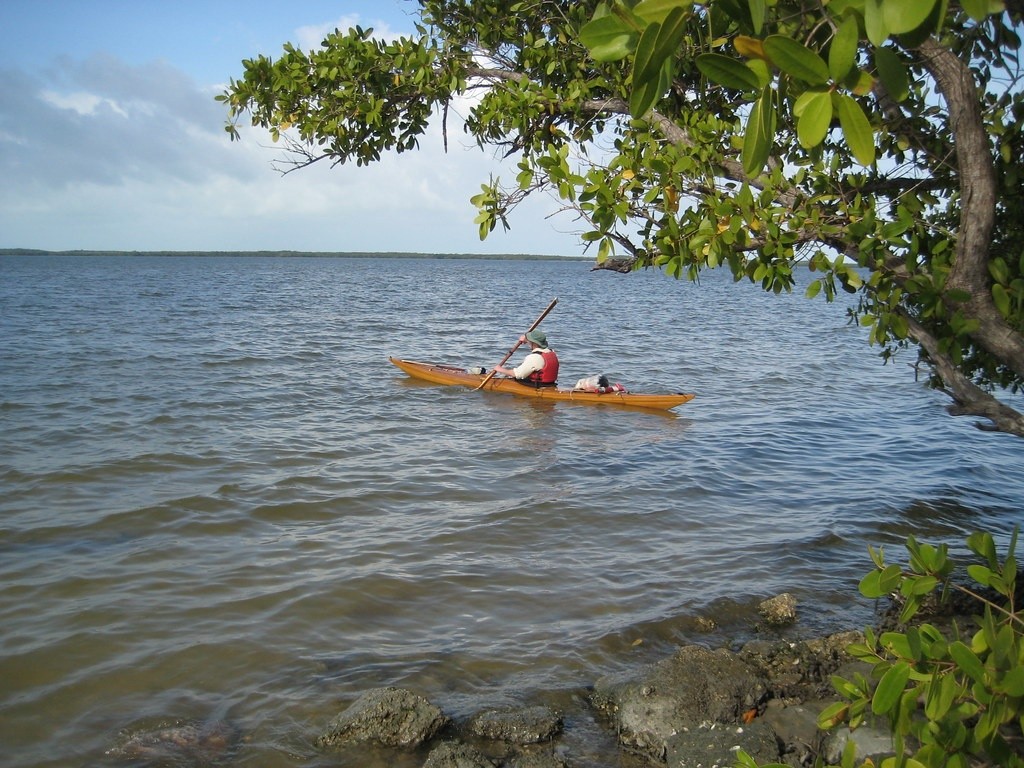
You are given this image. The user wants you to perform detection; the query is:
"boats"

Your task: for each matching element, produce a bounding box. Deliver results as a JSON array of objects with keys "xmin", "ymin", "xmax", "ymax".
[{"xmin": 388, "ymin": 355, "xmax": 697, "ymax": 411}]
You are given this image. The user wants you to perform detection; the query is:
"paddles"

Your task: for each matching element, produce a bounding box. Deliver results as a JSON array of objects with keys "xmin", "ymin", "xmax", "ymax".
[{"xmin": 474, "ymin": 297, "xmax": 559, "ymax": 390}]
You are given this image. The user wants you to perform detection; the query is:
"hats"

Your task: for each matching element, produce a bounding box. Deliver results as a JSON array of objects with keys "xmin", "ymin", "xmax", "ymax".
[{"xmin": 526, "ymin": 330, "xmax": 548, "ymax": 349}]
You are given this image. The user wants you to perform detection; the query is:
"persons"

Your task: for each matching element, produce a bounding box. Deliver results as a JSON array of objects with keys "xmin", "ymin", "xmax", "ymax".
[{"xmin": 493, "ymin": 330, "xmax": 559, "ymax": 384}]
